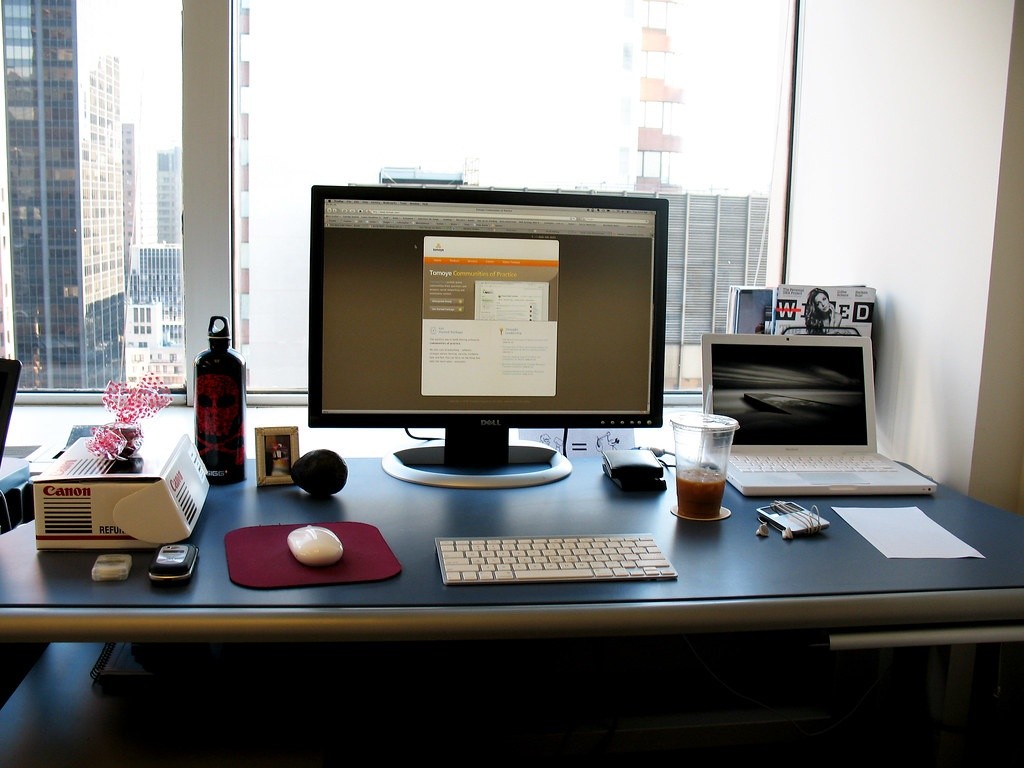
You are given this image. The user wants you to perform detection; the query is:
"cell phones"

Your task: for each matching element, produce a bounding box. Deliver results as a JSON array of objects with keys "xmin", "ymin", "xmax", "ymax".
[{"xmin": 149, "ymin": 544, "xmax": 199, "ymax": 588}]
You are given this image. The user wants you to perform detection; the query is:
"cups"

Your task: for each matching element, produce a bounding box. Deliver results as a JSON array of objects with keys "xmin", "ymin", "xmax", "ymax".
[{"xmin": 672, "ymin": 412, "xmax": 739, "ymax": 518}]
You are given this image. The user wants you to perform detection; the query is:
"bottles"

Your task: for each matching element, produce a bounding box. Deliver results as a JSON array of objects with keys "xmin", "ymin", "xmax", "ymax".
[{"xmin": 195, "ymin": 316, "xmax": 246, "ymax": 485}]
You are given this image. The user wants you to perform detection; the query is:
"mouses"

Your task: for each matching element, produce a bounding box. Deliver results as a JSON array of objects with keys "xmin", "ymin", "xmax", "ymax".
[{"xmin": 287, "ymin": 525, "xmax": 344, "ymax": 566}]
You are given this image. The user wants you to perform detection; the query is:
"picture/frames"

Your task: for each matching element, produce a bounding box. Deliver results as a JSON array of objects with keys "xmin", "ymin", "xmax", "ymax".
[{"xmin": 254, "ymin": 426, "xmax": 299, "ymax": 488}]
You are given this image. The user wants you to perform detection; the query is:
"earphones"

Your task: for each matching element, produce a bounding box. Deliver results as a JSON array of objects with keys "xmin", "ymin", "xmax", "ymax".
[
  {"xmin": 782, "ymin": 527, "xmax": 793, "ymax": 539},
  {"xmin": 755, "ymin": 524, "xmax": 770, "ymax": 536}
]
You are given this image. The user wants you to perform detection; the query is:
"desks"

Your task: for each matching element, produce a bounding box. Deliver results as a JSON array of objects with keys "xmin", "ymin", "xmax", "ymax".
[{"xmin": 0, "ymin": 455, "xmax": 1024, "ymax": 768}]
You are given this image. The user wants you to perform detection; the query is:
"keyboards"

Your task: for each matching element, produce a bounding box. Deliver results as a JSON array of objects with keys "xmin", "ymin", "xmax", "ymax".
[{"xmin": 435, "ymin": 533, "xmax": 678, "ymax": 587}]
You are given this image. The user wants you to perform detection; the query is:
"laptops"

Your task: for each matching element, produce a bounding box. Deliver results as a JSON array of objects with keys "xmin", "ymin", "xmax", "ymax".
[{"xmin": 701, "ymin": 334, "xmax": 938, "ymax": 496}]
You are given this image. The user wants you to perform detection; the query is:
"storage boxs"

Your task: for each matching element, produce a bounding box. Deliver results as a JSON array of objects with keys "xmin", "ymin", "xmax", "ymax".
[{"xmin": 32, "ymin": 432, "xmax": 210, "ymax": 551}]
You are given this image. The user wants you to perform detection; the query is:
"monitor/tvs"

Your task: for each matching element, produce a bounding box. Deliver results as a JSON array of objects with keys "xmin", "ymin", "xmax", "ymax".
[
  {"xmin": 0, "ymin": 358, "xmax": 23, "ymax": 465},
  {"xmin": 308, "ymin": 185, "xmax": 669, "ymax": 487}
]
